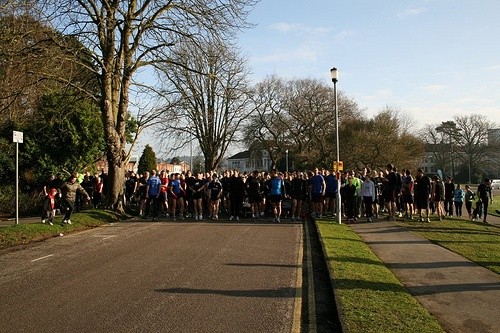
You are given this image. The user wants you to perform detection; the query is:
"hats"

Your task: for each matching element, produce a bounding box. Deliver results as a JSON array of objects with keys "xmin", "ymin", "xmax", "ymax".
[
  {"xmin": 70, "ymin": 172, "xmax": 77, "ymax": 178},
  {"xmin": 49, "ymin": 187, "xmax": 56, "ymax": 192}
]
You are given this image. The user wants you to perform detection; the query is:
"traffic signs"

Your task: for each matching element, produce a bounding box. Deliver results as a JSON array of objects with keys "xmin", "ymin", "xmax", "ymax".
[{"xmin": 12, "ymin": 130, "xmax": 23, "ymax": 144}]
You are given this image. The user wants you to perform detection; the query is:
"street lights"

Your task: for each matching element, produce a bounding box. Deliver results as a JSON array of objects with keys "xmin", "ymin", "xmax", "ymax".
[{"xmin": 330, "ymin": 67, "xmax": 343, "ymax": 224}]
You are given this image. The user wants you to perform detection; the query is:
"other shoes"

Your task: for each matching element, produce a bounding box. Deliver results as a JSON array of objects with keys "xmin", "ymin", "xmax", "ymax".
[
  {"xmin": 41, "ymin": 220, "xmax": 45, "ymax": 223},
  {"xmin": 194, "ymin": 215, "xmax": 198, "ymax": 221},
  {"xmin": 47, "ymin": 220, "xmax": 53, "ymax": 226},
  {"xmin": 228, "ymin": 216, "xmax": 234, "ymax": 221},
  {"xmin": 63, "ymin": 219, "xmax": 67, "ymax": 223},
  {"xmin": 236, "ymin": 216, "xmax": 240, "ymax": 221},
  {"xmin": 67, "ymin": 219, "xmax": 71, "ymax": 224},
  {"xmin": 199, "ymin": 214, "xmax": 203, "ymax": 220},
  {"xmin": 271, "ymin": 218, "xmax": 276, "ymax": 223},
  {"xmin": 277, "ymin": 218, "xmax": 281, "ymax": 223}
]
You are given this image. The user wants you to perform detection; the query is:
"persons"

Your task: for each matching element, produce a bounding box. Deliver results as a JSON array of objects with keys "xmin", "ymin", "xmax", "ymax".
[
  {"xmin": 56, "ymin": 174, "xmax": 90, "ymax": 224},
  {"xmin": 465, "ymin": 184, "xmax": 476, "ymax": 220},
  {"xmin": 41, "ymin": 188, "xmax": 57, "ymax": 225},
  {"xmin": 44, "ymin": 175, "xmax": 55, "ymax": 217},
  {"xmin": 72, "ymin": 164, "xmax": 455, "ymax": 222},
  {"xmin": 455, "ymin": 184, "xmax": 465, "ymax": 218},
  {"xmin": 477, "ymin": 179, "xmax": 494, "ymax": 224},
  {"xmin": 54, "ymin": 172, "xmax": 66, "ymax": 216}
]
